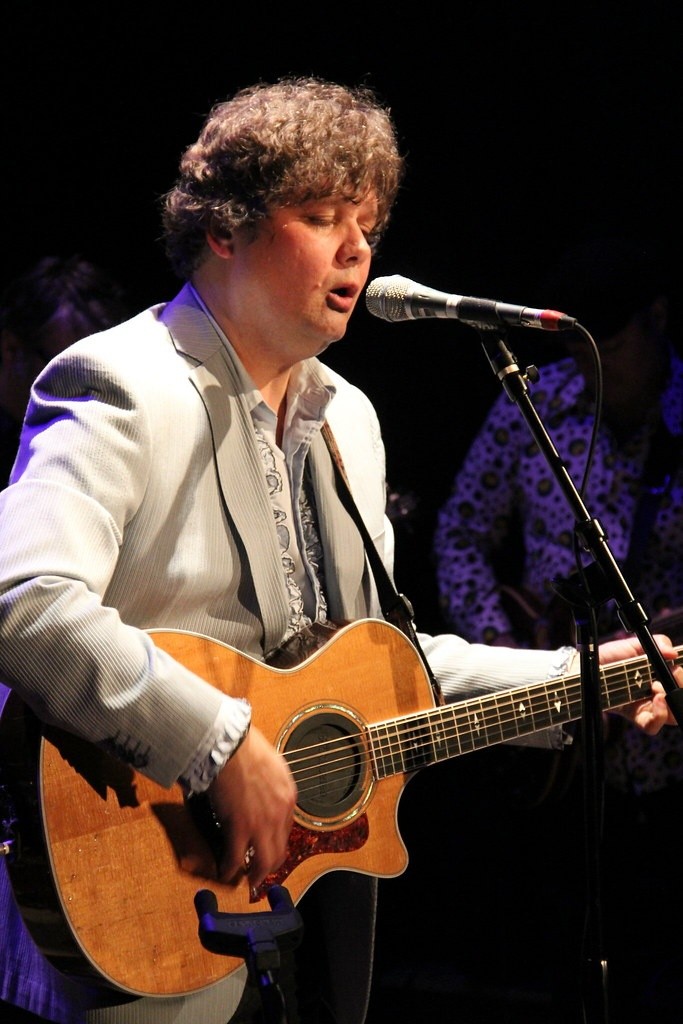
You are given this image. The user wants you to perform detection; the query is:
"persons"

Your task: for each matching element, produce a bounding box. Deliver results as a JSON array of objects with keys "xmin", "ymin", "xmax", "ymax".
[
  {"xmin": 0, "ymin": 77, "xmax": 683, "ymax": 1021},
  {"xmin": 0, "ymin": 251, "xmax": 141, "ymax": 488},
  {"xmin": 436, "ymin": 278, "xmax": 683, "ymax": 795}
]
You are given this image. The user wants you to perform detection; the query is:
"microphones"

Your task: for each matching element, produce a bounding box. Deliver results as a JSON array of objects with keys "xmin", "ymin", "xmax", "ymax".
[{"xmin": 366, "ymin": 274, "xmax": 577, "ymax": 331}]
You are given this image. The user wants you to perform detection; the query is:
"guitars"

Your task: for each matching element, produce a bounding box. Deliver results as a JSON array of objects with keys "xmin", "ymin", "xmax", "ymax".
[{"xmin": 2, "ymin": 614, "xmax": 683, "ymax": 999}]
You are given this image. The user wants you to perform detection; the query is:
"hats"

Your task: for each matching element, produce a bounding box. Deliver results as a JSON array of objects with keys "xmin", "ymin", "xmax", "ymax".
[{"xmin": 527, "ymin": 237, "xmax": 667, "ymax": 346}]
